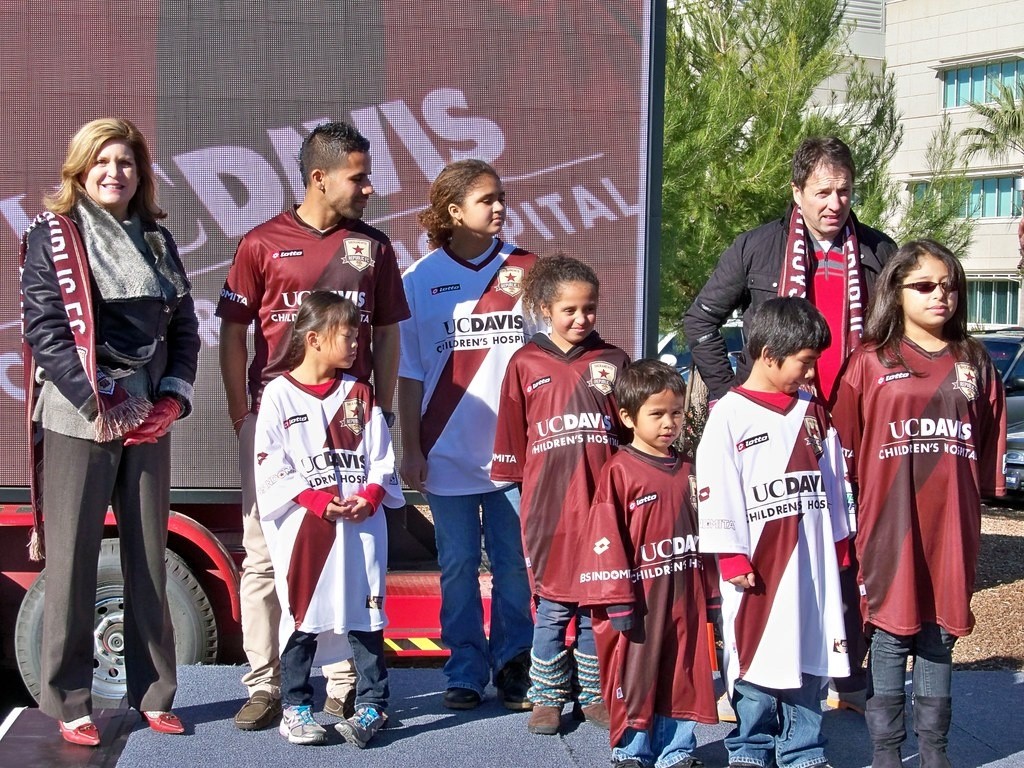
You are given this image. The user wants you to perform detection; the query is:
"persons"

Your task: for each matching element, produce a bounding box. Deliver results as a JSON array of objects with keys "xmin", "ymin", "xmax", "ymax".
[
  {"xmin": 398, "ymin": 156, "xmax": 543, "ymax": 712},
  {"xmin": 240, "ymin": 286, "xmax": 407, "ymax": 750},
  {"xmin": 828, "ymin": 235, "xmax": 1009, "ymax": 768},
  {"xmin": 217, "ymin": 119, "xmax": 416, "ymax": 731},
  {"xmin": 491, "ymin": 250, "xmax": 633, "ymax": 731},
  {"xmin": 571, "ymin": 359, "xmax": 721, "ymax": 768},
  {"xmin": 681, "ymin": 133, "xmax": 899, "ymax": 717},
  {"xmin": 19, "ymin": 111, "xmax": 201, "ymax": 746},
  {"xmin": 697, "ymin": 295, "xmax": 856, "ymax": 768}
]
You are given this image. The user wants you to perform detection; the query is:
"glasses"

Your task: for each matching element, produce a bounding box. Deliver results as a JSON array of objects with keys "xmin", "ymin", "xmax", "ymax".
[{"xmin": 895, "ymin": 281, "xmax": 959, "ymax": 292}]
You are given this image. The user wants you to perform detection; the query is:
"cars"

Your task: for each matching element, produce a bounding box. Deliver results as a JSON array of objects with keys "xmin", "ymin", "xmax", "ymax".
[
  {"xmin": 965, "ymin": 327, "xmax": 1024, "ymax": 498},
  {"xmin": 658, "ymin": 318, "xmax": 747, "ymax": 400}
]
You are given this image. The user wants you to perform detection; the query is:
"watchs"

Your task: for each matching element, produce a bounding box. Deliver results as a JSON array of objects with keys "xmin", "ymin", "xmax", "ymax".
[{"xmin": 383, "ymin": 411, "xmax": 397, "ymax": 426}]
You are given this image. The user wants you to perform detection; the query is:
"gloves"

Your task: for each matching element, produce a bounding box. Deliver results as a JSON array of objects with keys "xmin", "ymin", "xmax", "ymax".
[{"xmin": 123, "ymin": 396, "xmax": 182, "ymax": 446}]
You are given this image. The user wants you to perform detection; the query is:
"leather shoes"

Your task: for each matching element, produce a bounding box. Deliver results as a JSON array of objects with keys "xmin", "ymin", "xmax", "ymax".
[
  {"xmin": 58, "ymin": 719, "xmax": 101, "ymax": 745},
  {"xmin": 141, "ymin": 711, "xmax": 185, "ymax": 734}
]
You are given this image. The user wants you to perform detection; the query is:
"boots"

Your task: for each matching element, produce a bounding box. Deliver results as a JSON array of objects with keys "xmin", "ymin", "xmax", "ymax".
[
  {"xmin": 912, "ymin": 694, "xmax": 951, "ymax": 768},
  {"xmin": 865, "ymin": 691, "xmax": 908, "ymax": 767}
]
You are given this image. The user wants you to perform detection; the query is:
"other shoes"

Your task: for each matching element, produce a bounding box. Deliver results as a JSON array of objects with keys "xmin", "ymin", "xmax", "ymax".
[
  {"xmin": 528, "ymin": 705, "xmax": 561, "ymax": 734},
  {"xmin": 672, "ymin": 756, "xmax": 705, "ymax": 768},
  {"xmin": 827, "ymin": 688, "xmax": 870, "ymax": 713},
  {"xmin": 234, "ymin": 689, "xmax": 282, "ymax": 731},
  {"xmin": 497, "ymin": 682, "xmax": 533, "ymax": 709},
  {"xmin": 322, "ymin": 696, "xmax": 388, "ymax": 729},
  {"xmin": 610, "ymin": 758, "xmax": 645, "ymax": 768},
  {"xmin": 716, "ymin": 691, "xmax": 737, "ymax": 721},
  {"xmin": 444, "ymin": 686, "xmax": 479, "ymax": 708},
  {"xmin": 573, "ymin": 702, "xmax": 613, "ymax": 728}
]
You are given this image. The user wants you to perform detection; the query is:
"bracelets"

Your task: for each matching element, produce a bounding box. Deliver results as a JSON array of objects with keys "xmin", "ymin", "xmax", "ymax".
[{"xmin": 233, "ymin": 413, "xmax": 250, "ymax": 426}]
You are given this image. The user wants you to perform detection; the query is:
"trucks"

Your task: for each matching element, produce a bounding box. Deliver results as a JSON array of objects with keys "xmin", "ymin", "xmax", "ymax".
[{"xmin": 0, "ymin": 1, "xmax": 671, "ymax": 708}]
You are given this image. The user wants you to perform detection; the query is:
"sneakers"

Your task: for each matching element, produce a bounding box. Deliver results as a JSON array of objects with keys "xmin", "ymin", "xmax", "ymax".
[
  {"xmin": 279, "ymin": 704, "xmax": 328, "ymax": 745},
  {"xmin": 335, "ymin": 707, "xmax": 388, "ymax": 748}
]
